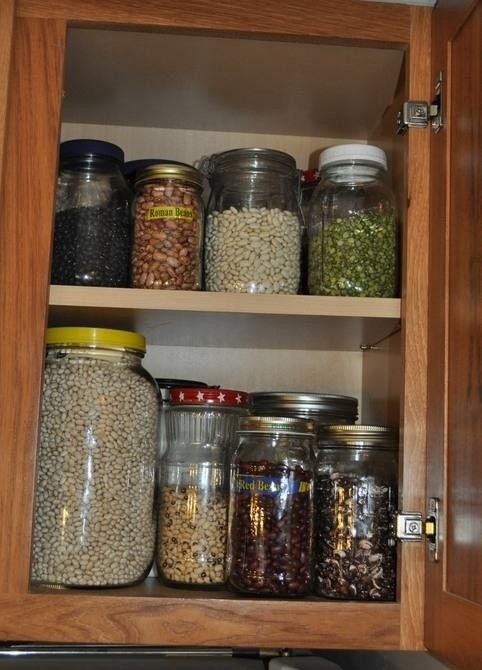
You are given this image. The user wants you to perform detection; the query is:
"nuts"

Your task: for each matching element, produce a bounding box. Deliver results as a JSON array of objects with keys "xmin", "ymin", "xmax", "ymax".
[
  {"xmin": 130, "ymin": 184, "xmax": 203, "ymax": 291},
  {"xmin": 203, "ymin": 206, "xmax": 304, "ymax": 296},
  {"xmin": 226, "ymin": 459, "xmax": 312, "ymax": 597},
  {"xmin": 29, "ymin": 355, "xmax": 158, "ymax": 586},
  {"xmin": 158, "ymin": 485, "xmax": 228, "ymax": 587},
  {"xmin": 312, "ymin": 471, "xmax": 397, "ymax": 600},
  {"xmin": 51, "ymin": 204, "xmax": 135, "ymax": 288},
  {"xmin": 306, "ymin": 209, "xmax": 396, "ymax": 297}
]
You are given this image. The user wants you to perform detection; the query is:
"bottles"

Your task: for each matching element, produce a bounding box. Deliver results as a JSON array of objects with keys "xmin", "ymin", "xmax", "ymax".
[
  {"xmin": 225, "ymin": 416, "xmax": 318, "ymax": 599},
  {"xmin": 206, "ymin": 146, "xmax": 306, "ymax": 296},
  {"xmin": 153, "ymin": 376, "xmax": 219, "ymax": 468},
  {"xmin": 24, "ymin": 325, "xmax": 162, "ymax": 590},
  {"xmin": 248, "ymin": 390, "xmax": 362, "ymax": 455},
  {"xmin": 50, "ymin": 137, "xmax": 132, "ymax": 288},
  {"xmin": 128, "ymin": 158, "xmax": 205, "ymax": 292},
  {"xmin": 313, "ymin": 424, "xmax": 397, "ymax": 601},
  {"xmin": 158, "ymin": 387, "xmax": 254, "ymax": 588},
  {"xmin": 302, "ymin": 142, "xmax": 401, "ymax": 298}
]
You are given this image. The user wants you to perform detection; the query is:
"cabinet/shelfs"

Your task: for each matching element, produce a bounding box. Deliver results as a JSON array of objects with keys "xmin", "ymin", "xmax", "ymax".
[{"xmin": 1, "ymin": 2, "xmax": 432, "ymax": 648}]
